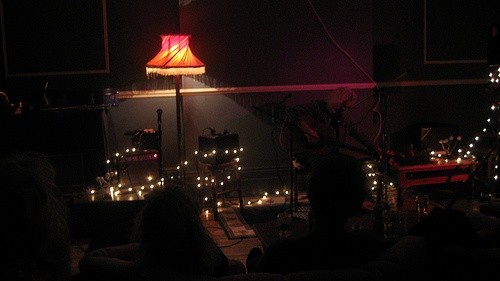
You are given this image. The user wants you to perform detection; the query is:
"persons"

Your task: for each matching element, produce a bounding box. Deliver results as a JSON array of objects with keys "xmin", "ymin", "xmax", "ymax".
[
  {"xmin": 130, "ymin": 184, "xmax": 231, "ymax": 278},
  {"xmin": 246, "ymin": 155, "xmax": 383, "ymax": 275},
  {"xmin": 281, "ymin": 88, "xmax": 382, "ymax": 171},
  {"xmin": 0, "ymin": 157, "xmax": 73, "ymax": 281}
]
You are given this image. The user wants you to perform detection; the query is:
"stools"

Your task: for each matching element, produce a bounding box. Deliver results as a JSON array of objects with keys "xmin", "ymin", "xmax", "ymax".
[
  {"xmin": 294, "ymin": 167, "xmax": 309, "ymax": 212},
  {"xmin": 198, "ymin": 158, "xmax": 245, "ymax": 222}
]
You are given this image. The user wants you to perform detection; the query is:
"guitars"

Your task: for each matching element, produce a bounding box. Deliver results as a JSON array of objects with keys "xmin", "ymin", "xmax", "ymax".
[{"xmin": 277, "ymin": 112, "xmax": 393, "ymax": 163}]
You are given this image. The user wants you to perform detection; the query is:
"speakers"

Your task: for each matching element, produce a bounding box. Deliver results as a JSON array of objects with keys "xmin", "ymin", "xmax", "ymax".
[
  {"xmin": 414, "ymin": 125, "xmax": 459, "ymax": 151},
  {"xmin": 69, "ymin": 200, "xmax": 122, "ymax": 239}
]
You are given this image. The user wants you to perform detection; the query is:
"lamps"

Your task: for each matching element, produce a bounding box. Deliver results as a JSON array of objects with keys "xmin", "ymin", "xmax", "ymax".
[{"xmin": 143, "ymin": 30, "xmax": 208, "ymax": 191}]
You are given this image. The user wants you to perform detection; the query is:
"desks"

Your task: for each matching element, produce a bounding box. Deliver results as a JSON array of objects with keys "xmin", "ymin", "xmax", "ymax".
[{"xmin": 373, "ymin": 155, "xmax": 479, "ymax": 234}]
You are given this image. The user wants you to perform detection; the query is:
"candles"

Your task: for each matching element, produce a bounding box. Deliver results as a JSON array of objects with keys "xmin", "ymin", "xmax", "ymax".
[{"xmin": 205, "ymin": 209, "xmax": 209, "ymax": 221}]
